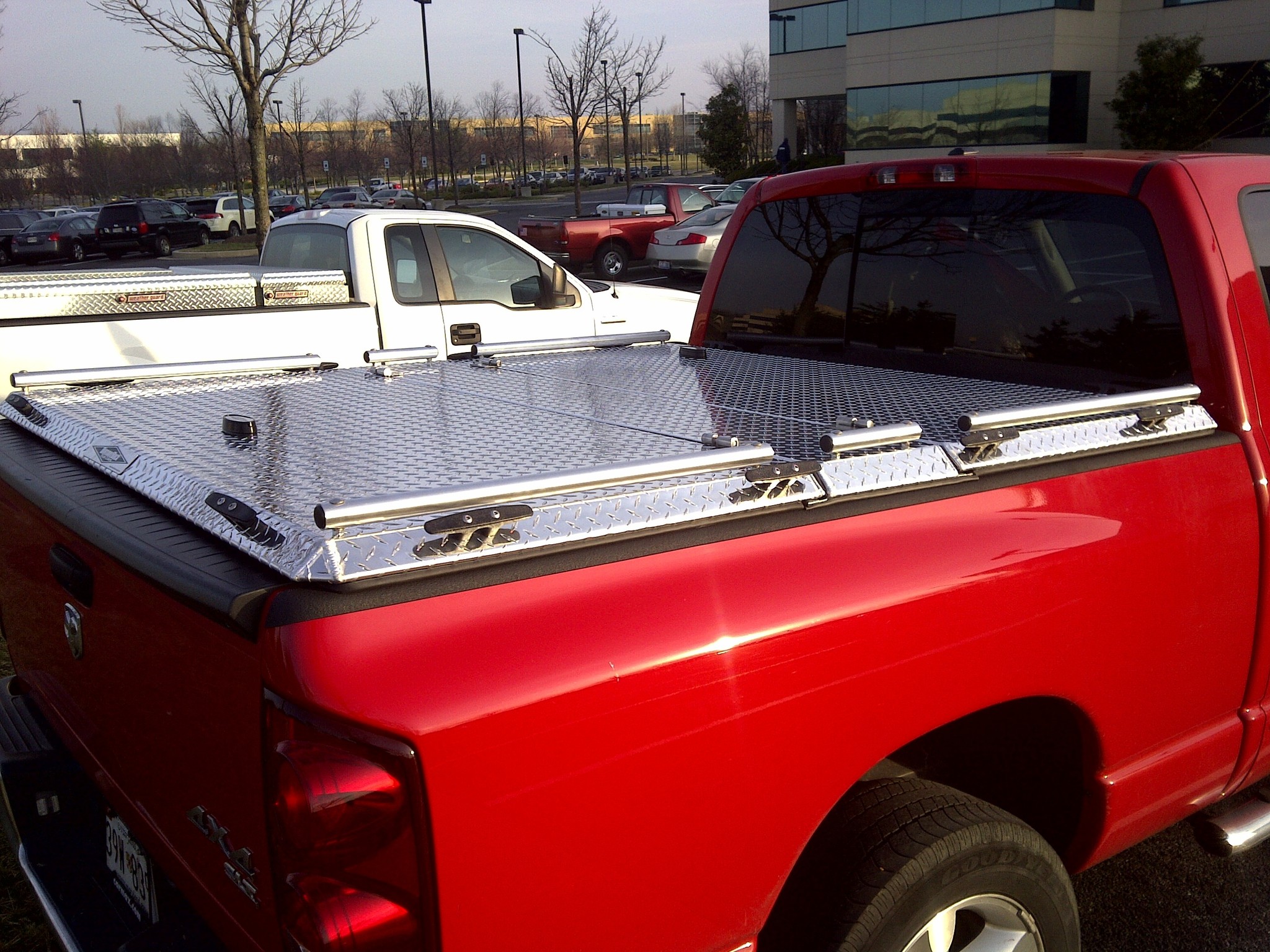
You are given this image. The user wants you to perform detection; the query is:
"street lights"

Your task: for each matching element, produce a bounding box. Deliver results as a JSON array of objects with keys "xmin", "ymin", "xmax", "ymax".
[
  {"xmin": 72, "ymin": 99, "xmax": 89, "ymax": 145},
  {"xmin": 635, "ymin": 72, "xmax": 643, "ymax": 172},
  {"xmin": 513, "ymin": 28, "xmax": 531, "ymax": 197},
  {"xmin": 680, "ymin": 92, "xmax": 686, "ymax": 175},
  {"xmin": 414, "ymin": 0, "xmax": 447, "ymax": 211},
  {"xmin": 600, "ymin": 60, "xmax": 615, "ymax": 184},
  {"xmin": 400, "ymin": 112, "xmax": 408, "ymax": 137},
  {"xmin": 272, "ymin": 100, "xmax": 288, "ymax": 195}
]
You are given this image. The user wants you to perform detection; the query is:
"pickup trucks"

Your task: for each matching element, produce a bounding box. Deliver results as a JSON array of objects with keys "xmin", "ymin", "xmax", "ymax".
[
  {"xmin": 517, "ymin": 182, "xmax": 716, "ymax": 281},
  {"xmin": 0, "ymin": 206, "xmax": 697, "ymax": 398},
  {"xmin": 0, "ymin": 149, "xmax": 1270, "ymax": 952}
]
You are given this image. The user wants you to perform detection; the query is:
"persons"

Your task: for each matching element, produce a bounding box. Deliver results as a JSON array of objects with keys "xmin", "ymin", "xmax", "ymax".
[{"xmin": 775, "ymin": 137, "xmax": 790, "ymax": 174}]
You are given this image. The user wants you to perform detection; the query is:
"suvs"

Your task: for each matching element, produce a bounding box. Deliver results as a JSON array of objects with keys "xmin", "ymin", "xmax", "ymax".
[
  {"xmin": 94, "ymin": 197, "xmax": 210, "ymax": 261},
  {"xmin": 365, "ymin": 178, "xmax": 401, "ymax": 192}
]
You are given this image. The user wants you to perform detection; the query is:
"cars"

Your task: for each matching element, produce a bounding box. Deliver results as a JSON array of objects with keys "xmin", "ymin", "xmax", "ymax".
[
  {"xmin": 371, "ymin": 189, "xmax": 426, "ymax": 210},
  {"xmin": 712, "ymin": 176, "xmax": 768, "ymax": 204},
  {"xmin": 0, "ymin": 205, "xmax": 102, "ymax": 268},
  {"xmin": 268, "ymin": 195, "xmax": 321, "ymax": 220},
  {"xmin": 312, "ymin": 186, "xmax": 371, "ymax": 208},
  {"xmin": 183, "ymin": 196, "xmax": 276, "ymax": 239},
  {"xmin": 648, "ymin": 184, "xmax": 742, "ymax": 206},
  {"xmin": 10, "ymin": 215, "xmax": 104, "ymax": 266},
  {"xmin": 321, "ymin": 191, "xmax": 384, "ymax": 209},
  {"xmin": 423, "ymin": 164, "xmax": 672, "ymax": 189},
  {"xmin": 267, "ymin": 189, "xmax": 287, "ymax": 198},
  {"xmin": 644, "ymin": 203, "xmax": 739, "ymax": 288},
  {"xmin": 165, "ymin": 192, "xmax": 254, "ymax": 210}
]
